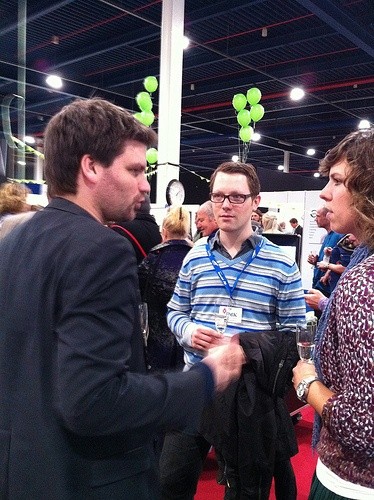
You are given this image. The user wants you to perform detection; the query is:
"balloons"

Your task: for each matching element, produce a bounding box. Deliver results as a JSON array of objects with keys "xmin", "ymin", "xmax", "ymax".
[
  {"xmin": 239, "ymin": 125, "xmax": 253, "ymax": 142},
  {"xmin": 146, "ymin": 147, "xmax": 158, "ymax": 164},
  {"xmin": 144, "ymin": 76, "xmax": 158, "ymax": 92},
  {"xmin": 136, "ymin": 92, "xmax": 153, "ymax": 112},
  {"xmin": 135, "ymin": 110, "xmax": 154, "ymax": 127},
  {"xmin": 237, "ymin": 109, "xmax": 250, "ymax": 126},
  {"xmin": 232, "ymin": 93, "xmax": 247, "ymax": 112},
  {"xmin": 250, "ymin": 104, "xmax": 264, "ymax": 122},
  {"xmin": 247, "ymin": 87, "xmax": 261, "ymax": 105}
]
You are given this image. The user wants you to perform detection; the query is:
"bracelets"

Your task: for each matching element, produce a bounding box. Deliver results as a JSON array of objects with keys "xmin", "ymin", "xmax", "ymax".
[{"xmin": 325, "ymin": 262, "xmax": 329, "ymax": 268}]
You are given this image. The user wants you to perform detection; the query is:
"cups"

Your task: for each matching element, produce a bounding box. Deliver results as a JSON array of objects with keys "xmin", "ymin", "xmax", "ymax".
[
  {"xmin": 215, "ymin": 301, "xmax": 228, "ymax": 334},
  {"xmin": 139, "ymin": 304, "xmax": 149, "ymax": 343},
  {"xmin": 296, "ymin": 321, "xmax": 317, "ymax": 364}
]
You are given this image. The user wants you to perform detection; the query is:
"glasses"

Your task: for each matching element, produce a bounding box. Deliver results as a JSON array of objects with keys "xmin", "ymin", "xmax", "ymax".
[{"xmin": 209, "ymin": 193, "xmax": 253, "ymax": 204}]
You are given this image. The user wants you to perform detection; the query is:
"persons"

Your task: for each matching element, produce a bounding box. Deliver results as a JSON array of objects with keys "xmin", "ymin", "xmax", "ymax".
[
  {"xmin": 261, "ymin": 210, "xmax": 282, "ymax": 233},
  {"xmin": 106, "ymin": 161, "xmax": 308, "ymax": 500},
  {"xmin": 291, "ymin": 126, "xmax": 374, "ymax": 500},
  {"xmin": 0, "ymin": 183, "xmax": 38, "ymax": 241},
  {"xmin": 290, "ymin": 218, "xmax": 303, "ymax": 235},
  {"xmin": 0, "ymin": 97, "xmax": 243, "ymax": 500},
  {"xmin": 280, "ymin": 220, "xmax": 286, "ymax": 232},
  {"xmin": 252, "ymin": 209, "xmax": 264, "ymax": 232}
]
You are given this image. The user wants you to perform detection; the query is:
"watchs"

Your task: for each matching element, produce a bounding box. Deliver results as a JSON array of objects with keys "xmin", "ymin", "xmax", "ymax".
[{"xmin": 296, "ymin": 376, "xmax": 320, "ymax": 403}]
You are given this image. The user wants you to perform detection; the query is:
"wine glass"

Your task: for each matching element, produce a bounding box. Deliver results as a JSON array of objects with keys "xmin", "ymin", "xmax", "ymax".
[{"xmin": 310, "ymin": 250, "xmax": 317, "ymax": 269}]
